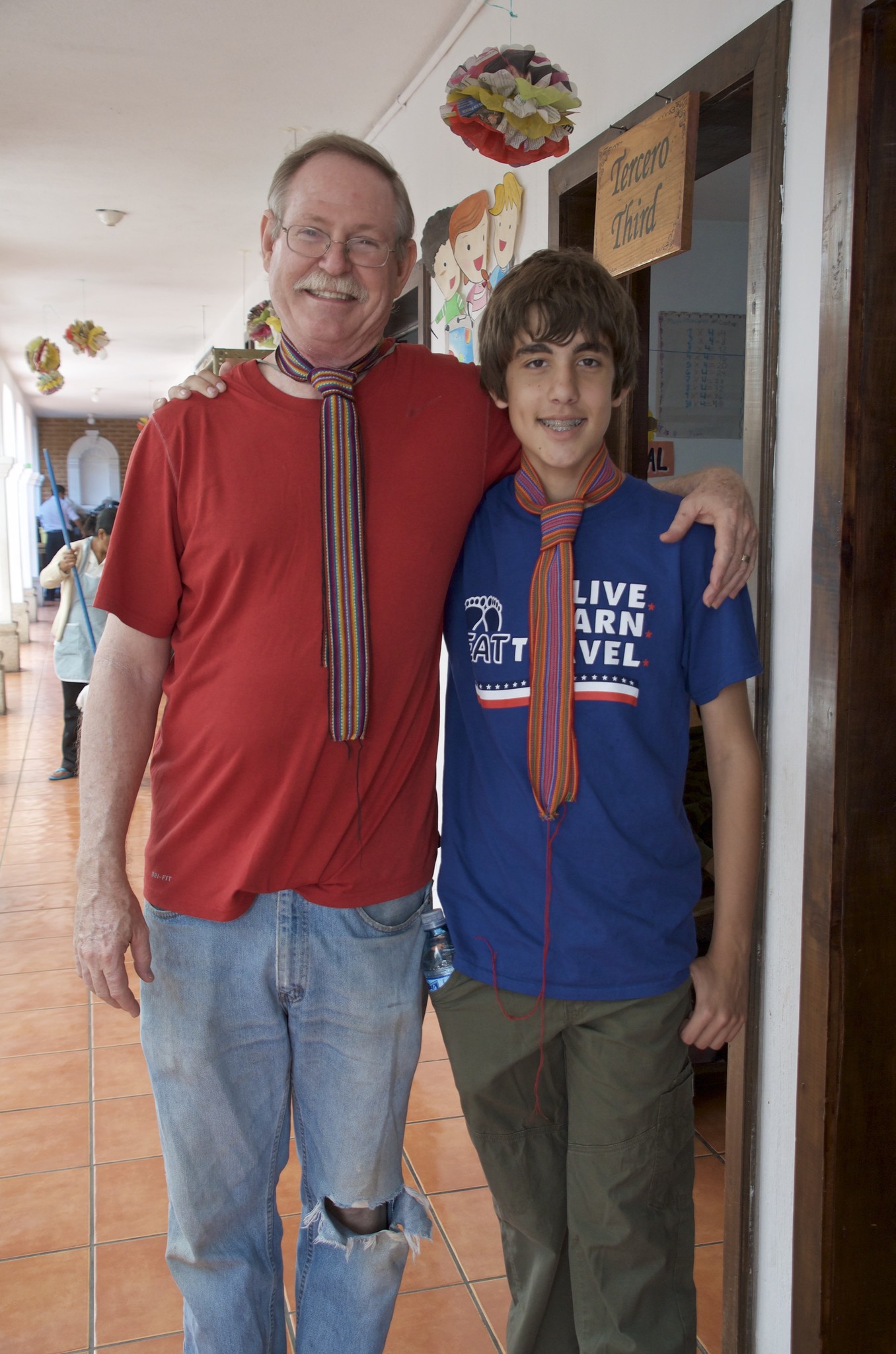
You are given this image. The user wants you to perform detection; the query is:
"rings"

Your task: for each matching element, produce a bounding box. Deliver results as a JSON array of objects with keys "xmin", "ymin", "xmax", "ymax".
[{"xmin": 740, "ymin": 552, "xmax": 751, "ymax": 565}]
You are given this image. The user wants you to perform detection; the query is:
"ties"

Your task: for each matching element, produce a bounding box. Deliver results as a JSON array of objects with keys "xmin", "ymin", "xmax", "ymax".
[
  {"xmin": 513, "ymin": 439, "xmax": 622, "ymax": 820},
  {"xmin": 272, "ymin": 328, "xmax": 393, "ymax": 742}
]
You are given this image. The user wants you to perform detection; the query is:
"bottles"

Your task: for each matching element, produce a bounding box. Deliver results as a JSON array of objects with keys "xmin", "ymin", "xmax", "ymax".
[{"xmin": 421, "ymin": 908, "xmax": 454, "ymax": 992}]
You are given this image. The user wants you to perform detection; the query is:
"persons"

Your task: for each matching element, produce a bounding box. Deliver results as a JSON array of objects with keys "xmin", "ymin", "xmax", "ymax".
[
  {"xmin": 73, "ymin": 135, "xmax": 760, "ymax": 1354},
  {"xmin": 423, "ymin": 244, "xmax": 769, "ymax": 1354},
  {"xmin": 32, "ymin": 482, "xmax": 122, "ymax": 782}
]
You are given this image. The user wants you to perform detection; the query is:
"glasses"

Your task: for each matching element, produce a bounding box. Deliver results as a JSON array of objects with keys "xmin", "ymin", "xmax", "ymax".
[{"xmin": 270, "ymin": 212, "xmax": 403, "ymax": 270}]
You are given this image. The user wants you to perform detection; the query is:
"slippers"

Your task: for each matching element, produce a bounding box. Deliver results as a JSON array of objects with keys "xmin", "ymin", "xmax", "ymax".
[{"xmin": 48, "ymin": 769, "xmax": 73, "ymax": 780}]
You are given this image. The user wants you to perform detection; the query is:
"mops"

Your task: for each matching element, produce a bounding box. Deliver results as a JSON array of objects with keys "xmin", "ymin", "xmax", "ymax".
[{"xmin": 42, "ymin": 443, "xmax": 98, "ymax": 711}]
